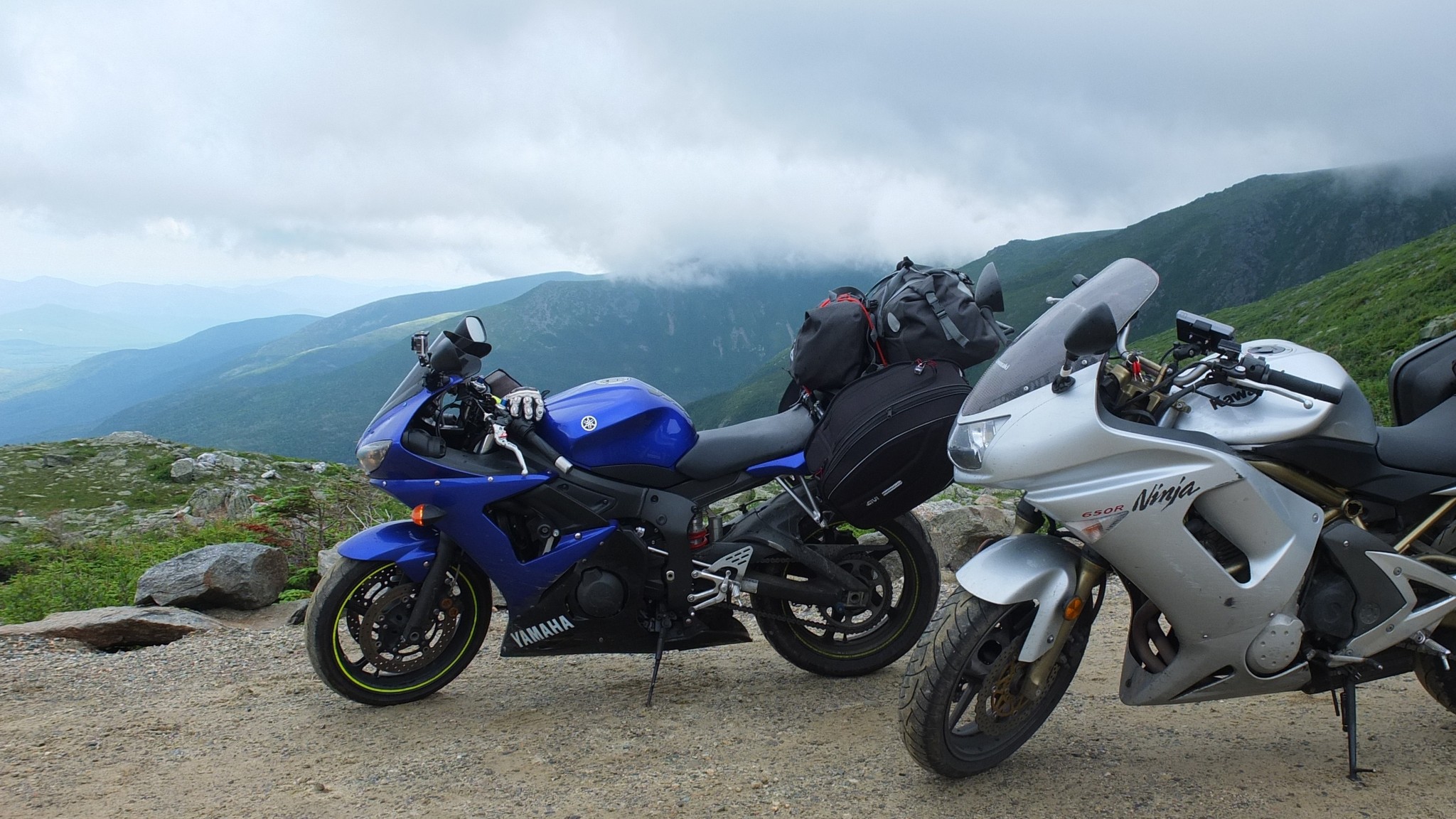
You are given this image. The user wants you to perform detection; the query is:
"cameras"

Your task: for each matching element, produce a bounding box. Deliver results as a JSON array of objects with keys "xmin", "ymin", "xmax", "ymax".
[
  {"xmin": 415, "ymin": 335, "xmax": 429, "ymax": 357},
  {"xmin": 1175, "ymin": 310, "xmax": 1234, "ymax": 353}
]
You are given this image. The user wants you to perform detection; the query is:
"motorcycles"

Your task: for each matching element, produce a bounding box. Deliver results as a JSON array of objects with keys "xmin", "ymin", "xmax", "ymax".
[
  {"xmin": 891, "ymin": 252, "xmax": 1455, "ymax": 790},
  {"xmin": 303, "ymin": 314, "xmax": 1016, "ymax": 709}
]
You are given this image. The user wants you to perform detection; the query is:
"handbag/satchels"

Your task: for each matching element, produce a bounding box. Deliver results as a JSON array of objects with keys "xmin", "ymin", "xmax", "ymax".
[
  {"xmin": 803, "ymin": 358, "xmax": 974, "ymax": 531},
  {"xmin": 866, "ymin": 256, "xmax": 1001, "ymax": 370}
]
